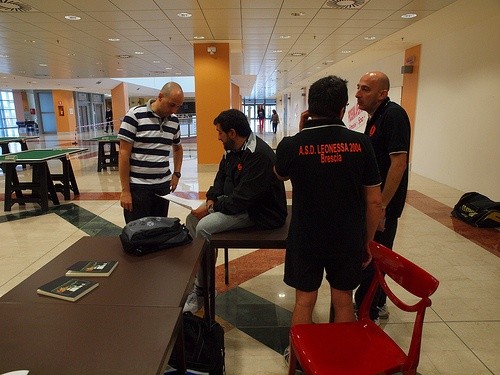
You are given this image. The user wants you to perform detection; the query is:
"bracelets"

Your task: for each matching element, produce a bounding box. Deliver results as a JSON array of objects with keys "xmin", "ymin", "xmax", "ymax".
[{"xmin": 382, "ymin": 207, "xmax": 386, "ymax": 208}]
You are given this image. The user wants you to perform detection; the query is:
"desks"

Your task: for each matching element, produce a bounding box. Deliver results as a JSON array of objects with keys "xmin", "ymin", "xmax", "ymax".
[
  {"xmin": 0, "ymin": 302, "xmax": 182, "ymax": 375},
  {"xmin": 207, "ymin": 203, "xmax": 334, "ymax": 323},
  {"xmin": 0, "ymin": 233, "xmax": 206, "ymax": 311},
  {"xmin": 82, "ymin": 136, "xmax": 120, "ymax": 172},
  {"xmin": 0, "ymin": 147, "xmax": 89, "ymax": 212},
  {"xmin": 0, "ymin": 136, "xmax": 39, "ymax": 168}
]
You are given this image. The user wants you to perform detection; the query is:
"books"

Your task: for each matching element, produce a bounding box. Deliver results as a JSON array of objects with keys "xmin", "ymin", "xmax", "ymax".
[
  {"xmin": 36, "ymin": 275, "xmax": 99, "ymax": 302},
  {"xmin": 65, "ymin": 260, "xmax": 120, "ymax": 277}
]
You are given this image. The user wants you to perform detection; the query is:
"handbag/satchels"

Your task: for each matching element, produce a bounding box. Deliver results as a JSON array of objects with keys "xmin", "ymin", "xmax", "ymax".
[{"xmin": 452, "ymin": 192, "xmax": 500, "ymax": 230}]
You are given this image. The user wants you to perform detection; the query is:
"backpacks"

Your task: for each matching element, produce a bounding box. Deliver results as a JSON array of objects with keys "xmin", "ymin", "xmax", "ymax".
[
  {"xmin": 119, "ymin": 217, "xmax": 194, "ymax": 257},
  {"xmin": 168, "ymin": 311, "xmax": 225, "ymax": 375}
]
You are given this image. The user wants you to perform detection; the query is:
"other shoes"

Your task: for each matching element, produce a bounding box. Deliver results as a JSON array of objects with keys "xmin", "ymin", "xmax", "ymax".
[
  {"xmin": 354, "ymin": 310, "xmax": 381, "ymax": 326},
  {"xmin": 283, "ymin": 347, "xmax": 304, "ymax": 373},
  {"xmin": 352, "ymin": 301, "xmax": 389, "ymax": 318}
]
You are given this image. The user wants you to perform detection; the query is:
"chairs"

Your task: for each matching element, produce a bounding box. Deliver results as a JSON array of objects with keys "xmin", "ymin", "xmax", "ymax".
[{"xmin": 286, "ymin": 240, "xmax": 438, "ymax": 375}]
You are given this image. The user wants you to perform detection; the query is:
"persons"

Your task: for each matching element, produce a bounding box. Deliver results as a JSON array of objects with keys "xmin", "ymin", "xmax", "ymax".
[
  {"xmin": 183, "ymin": 108, "xmax": 288, "ymax": 313},
  {"xmin": 273, "ymin": 75, "xmax": 382, "ymax": 373},
  {"xmin": 257, "ymin": 105, "xmax": 266, "ymax": 134},
  {"xmin": 117, "ymin": 82, "xmax": 184, "ymax": 225},
  {"xmin": 270, "ymin": 110, "xmax": 280, "ymax": 134},
  {"xmin": 352, "ymin": 71, "xmax": 411, "ymax": 326},
  {"xmin": 103, "ymin": 105, "xmax": 114, "ymax": 133}
]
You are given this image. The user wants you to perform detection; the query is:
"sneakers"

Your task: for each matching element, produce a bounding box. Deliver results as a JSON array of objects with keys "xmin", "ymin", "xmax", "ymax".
[{"xmin": 183, "ymin": 289, "xmax": 217, "ymax": 313}]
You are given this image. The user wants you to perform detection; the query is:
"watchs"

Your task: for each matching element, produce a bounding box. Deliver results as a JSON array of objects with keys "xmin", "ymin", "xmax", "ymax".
[
  {"xmin": 173, "ymin": 172, "xmax": 181, "ymax": 178},
  {"xmin": 208, "ymin": 204, "xmax": 214, "ymax": 214}
]
